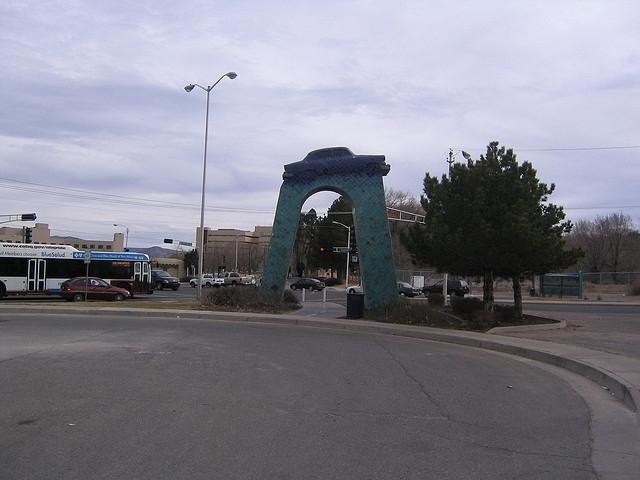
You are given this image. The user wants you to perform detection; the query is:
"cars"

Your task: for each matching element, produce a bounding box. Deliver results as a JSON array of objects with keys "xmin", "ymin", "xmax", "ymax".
[
  {"xmin": 397, "ymin": 282, "xmax": 420, "ymax": 297},
  {"xmin": 346, "ymin": 282, "xmax": 364, "ymax": 294},
  {"xmin": 190, "ymin": 272, "xmax": 256, "ymax": 288},
  {"xmin": 58, "ymin": 277, "xmax": 132, "ymax": 301},
  {"xmin": 290, "ymin": 278, "xmax": 325, "ymax": 291}
]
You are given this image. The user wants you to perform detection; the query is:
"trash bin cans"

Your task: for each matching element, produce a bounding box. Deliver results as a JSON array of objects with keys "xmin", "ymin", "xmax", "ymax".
[{"xmin": 345, "ymin": 293, "xmax": 365, "ymax": 320}]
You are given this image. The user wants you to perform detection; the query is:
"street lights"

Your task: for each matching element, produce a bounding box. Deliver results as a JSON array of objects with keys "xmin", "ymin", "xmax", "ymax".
[
  {"xmin": 113, "ymin": 223, "xmax": 128, "ymax": 249},
  {"xmin": 184, "ymin": 72, "xmax": 238, "ymax": 300},
  {"xmin": 332, "ymin": 221, "xmax": 350, "ymax": 288}
]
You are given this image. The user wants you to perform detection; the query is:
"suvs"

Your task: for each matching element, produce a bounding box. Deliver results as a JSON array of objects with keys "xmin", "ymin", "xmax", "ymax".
[
  {"xmin": 151, "ymin": 270, "xmax": 181, "ymax": 291},
  {"xmin": 423, "ymin": 279, "xmax": 469, "ymax": 298}
]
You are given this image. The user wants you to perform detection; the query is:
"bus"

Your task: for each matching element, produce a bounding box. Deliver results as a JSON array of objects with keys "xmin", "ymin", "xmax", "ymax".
[{"xmin": 0, "ymin": 243, "xmax": 154, "ymax": 299}]
